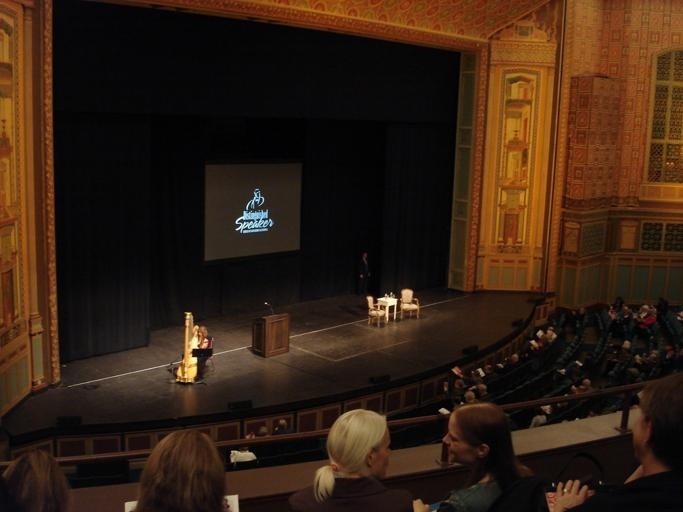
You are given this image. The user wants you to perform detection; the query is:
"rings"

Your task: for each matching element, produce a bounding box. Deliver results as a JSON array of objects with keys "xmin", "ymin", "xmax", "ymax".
[{"xmin": 563, "ymin": 488, "xmax": 570, "ymax": 492}]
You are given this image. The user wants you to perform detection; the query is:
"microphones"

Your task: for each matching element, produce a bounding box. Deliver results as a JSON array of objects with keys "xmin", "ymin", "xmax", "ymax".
[{"xmin": 264, "ymin": 302, "xmax": 274, "ymax": 315}]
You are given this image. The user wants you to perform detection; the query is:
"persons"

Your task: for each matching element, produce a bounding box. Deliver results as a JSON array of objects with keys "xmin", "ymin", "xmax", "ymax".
[
  {"xmin": 443, "ymin": 297, "xmax": 683, "ymax": 429},
  {"xmin": 554, "ymin": 372, "xmax": 683, "ymax": 511},
  {"xmin": 0, "ymin": 449, "xmax": 70, "ymax": 512},
  {"xmin": 285, "ymin": 409, "xmax": 414, "ymax": 512},
  {"xmin": 198, "ymin": 326, "xmax": 210, "ymax": 349},
  {"xmin": 130, "ymin": 430, "xmax": 233, "ymax": 512},
  {"xmin": 414, "ymin": 402, "xmax": 548, "ymax": 511}
]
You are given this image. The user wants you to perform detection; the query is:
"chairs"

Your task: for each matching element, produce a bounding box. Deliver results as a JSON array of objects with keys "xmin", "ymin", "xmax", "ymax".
[{"xmin": 1, "ymin": 285, "xmax": 682, "ymax": 512}]
